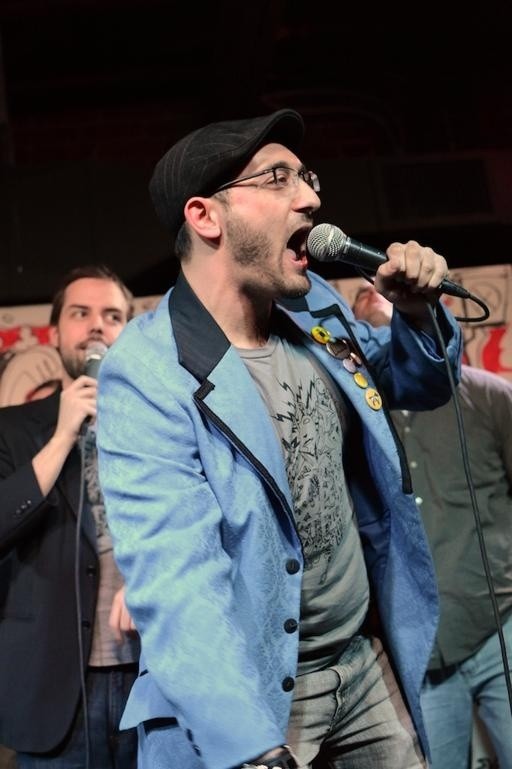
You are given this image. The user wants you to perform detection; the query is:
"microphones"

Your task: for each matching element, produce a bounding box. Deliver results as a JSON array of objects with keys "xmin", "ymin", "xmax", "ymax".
[
  {"xmin": 306, "ymin": 223, "xmax": 472, "ymax": 300},
  {"xmin": 80, "ymin": 341, "xmax": 109, "ymax": 437}
]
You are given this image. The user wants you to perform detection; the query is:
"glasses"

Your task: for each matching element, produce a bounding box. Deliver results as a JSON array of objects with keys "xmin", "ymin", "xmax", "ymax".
[{"xmin": 209, "ymin": 166, "xmax": 323, "ymax": 193}]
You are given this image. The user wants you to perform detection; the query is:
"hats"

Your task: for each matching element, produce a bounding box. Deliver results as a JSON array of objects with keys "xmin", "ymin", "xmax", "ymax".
[{"xmin": 149, "ymin": 108, "xmax": 306, "ymax": 237}]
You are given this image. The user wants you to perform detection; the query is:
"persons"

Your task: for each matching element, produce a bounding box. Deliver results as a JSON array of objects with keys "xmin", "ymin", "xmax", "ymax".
[
  {"xmin": 0, "ymin": 262, "xmax": 124, "ymax": 769},
  {"xmin": 91, "ymin": 107, "xmax": 447, "ymax": 769},
  {"xmin": 351, "ymin": 285, "xmax": 512, "ymax": 769},
  {"xmin": 0, "ymin": 345, "xmax": 64, "ymax": 409}
]
